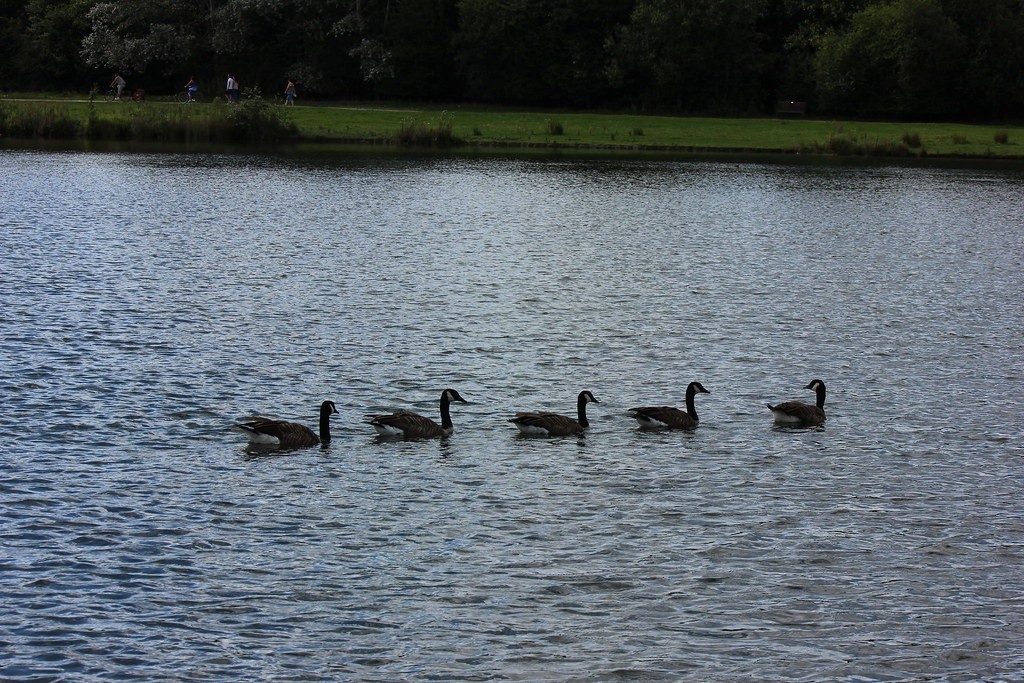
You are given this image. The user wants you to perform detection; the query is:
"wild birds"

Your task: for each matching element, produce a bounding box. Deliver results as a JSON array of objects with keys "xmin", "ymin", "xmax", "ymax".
[
  {"xmin": 623, "ymin": 381, "xmax": 710, "ymax": 431},
  {"xmin": 361, "ymin": 390, "xmax": 465, "ymax": 441},
  {"xmin": 767, "ymin": 378, "xmax": 826, "ymax": 425},
  {"xmin": 233, "ymin": 399, "xmax": 339, "ymax": 456},
  {"xmin": 508, "ymin": 391, "xmax": 600, "ymax": 438}
]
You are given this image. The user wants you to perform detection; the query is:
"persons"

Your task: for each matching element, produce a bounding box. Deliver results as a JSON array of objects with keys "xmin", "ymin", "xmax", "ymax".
[
  {"xmin": 284, "ymin": 79, "xmax": 296, "ymax": 107},
  {"xmin": 224, "ymin": 73, "xmax": 239, "ymax": 105},
  {"xmin": 111, "ymin": 73, "xmax": 126, "ymax": 99},
  {"xmin": 184, "ymin": 76, "xmax": 198, "ymax": 103}
]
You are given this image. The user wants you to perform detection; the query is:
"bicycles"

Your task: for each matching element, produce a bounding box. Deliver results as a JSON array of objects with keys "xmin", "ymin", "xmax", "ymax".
[
  {"xmin": 178, "ymin": 90, "xmax": 203, "ymax": 104},
  {"xmin": 105, "ymin": 85, "xmax": 131, "ymax": 102}
]
